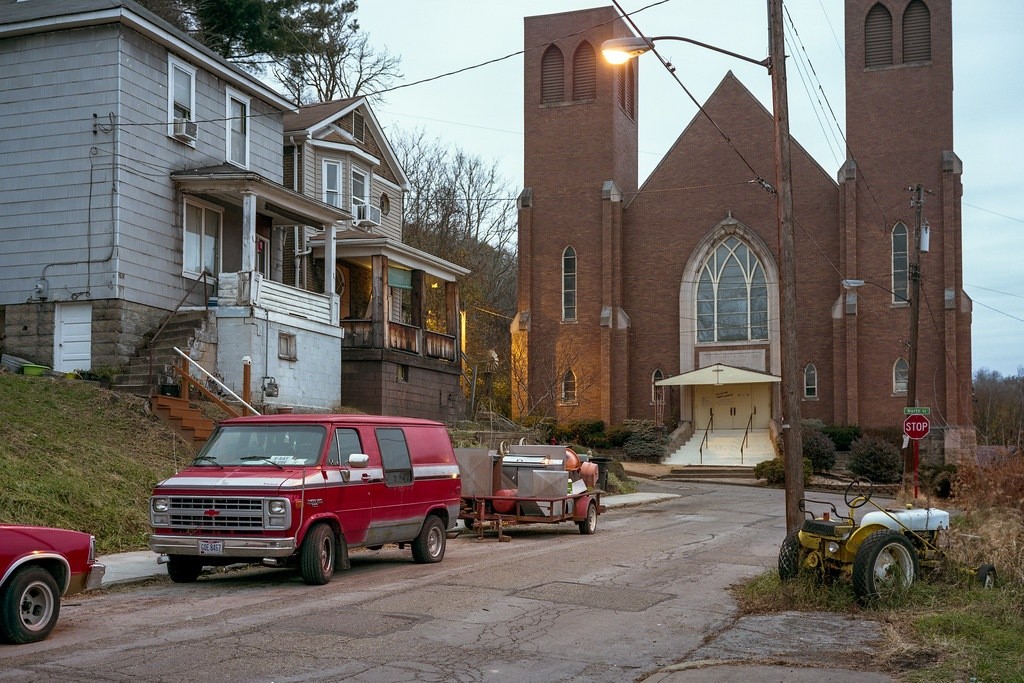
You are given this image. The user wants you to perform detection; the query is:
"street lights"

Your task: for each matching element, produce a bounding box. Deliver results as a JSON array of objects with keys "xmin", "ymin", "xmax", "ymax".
[
  {"xmin": 601, "ymin": 37, "xmax": 806, "ymax": 534},
  {"xmin": 841, "ymin": 280, "xmax": 918, "ymax": 494}
]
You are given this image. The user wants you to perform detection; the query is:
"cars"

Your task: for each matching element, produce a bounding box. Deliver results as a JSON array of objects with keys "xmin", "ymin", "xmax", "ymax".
[{"xmin": 0, "ymin": 525, "xmax": 107, "ymax": 644}]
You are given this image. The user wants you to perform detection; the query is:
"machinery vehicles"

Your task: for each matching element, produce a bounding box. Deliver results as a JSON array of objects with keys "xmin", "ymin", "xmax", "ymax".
[{"xmin": 778, "ymin": 477, "xmax": 997, "ymax": 612}]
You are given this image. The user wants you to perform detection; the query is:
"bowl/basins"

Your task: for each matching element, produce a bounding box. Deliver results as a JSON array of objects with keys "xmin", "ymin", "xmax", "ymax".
[
  {"xmin": 22, "ymin": 364, "xmax": 50, "ymax": 376},
  {"xmin": 1, "ymin": 354, "xmax": 33, "ymax": 374},
  {"xmin": 65, "ymin": 373, "xmax": 78, "ymax": 379},
  {"xmin": 566, "ymin": 448, "xmax": 580, "ymax": 471}
]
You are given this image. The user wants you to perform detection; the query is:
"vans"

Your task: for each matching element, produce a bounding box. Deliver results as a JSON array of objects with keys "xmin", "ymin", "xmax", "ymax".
[{"xmin": 150, "ymin": 415, "xmax": 462, "ymax": 585}]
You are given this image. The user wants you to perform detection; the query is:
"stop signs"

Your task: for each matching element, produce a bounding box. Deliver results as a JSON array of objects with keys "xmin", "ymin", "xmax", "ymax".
[{"xmin": 904, "ymin": 415, "xmax": 930, "ymax": 439}]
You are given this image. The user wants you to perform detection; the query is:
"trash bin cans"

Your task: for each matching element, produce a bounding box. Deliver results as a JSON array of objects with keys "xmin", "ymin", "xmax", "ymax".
[
  {"xmin": 589, "ymin": 456, "xmax": 613, "ymax": 491},
  {"xmin": 578, "ymin": 454, "xmax": 589, "ymax": 477}
]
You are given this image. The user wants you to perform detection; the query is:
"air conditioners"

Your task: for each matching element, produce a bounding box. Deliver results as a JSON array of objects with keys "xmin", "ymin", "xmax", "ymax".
[
  {"xmin": 358, "ymin": 205, "xmax": 381, "ymax": 226},
  {"xmin": 174, "ymin": 118, "xmax": 198, "ymax": 141}
]
